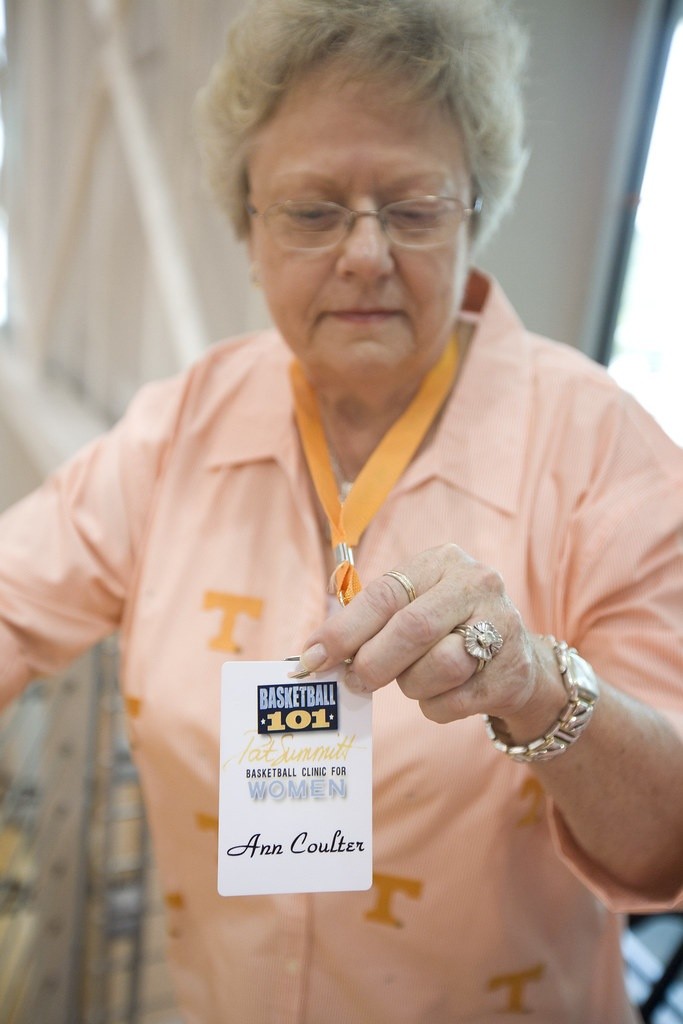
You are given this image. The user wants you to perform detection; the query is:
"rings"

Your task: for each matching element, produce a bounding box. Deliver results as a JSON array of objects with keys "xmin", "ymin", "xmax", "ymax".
[
  {"xmin": 449, "ymin": 620, "xmax": 504, "ymax": 676},
  {"xmin": 383, "ymin": 569, "xmax": 416, "ymax": 603}
]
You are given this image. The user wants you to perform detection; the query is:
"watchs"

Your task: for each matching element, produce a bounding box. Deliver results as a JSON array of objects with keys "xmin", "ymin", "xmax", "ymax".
[{"xmin": 484, "ymin": 634, "xmax": 600, "ymax": 764}]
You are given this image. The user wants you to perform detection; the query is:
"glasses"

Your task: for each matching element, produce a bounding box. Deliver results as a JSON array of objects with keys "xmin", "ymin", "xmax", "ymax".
[{"xmin": 241, "ymin": 196, "xmax": 481, "ymax": 253}]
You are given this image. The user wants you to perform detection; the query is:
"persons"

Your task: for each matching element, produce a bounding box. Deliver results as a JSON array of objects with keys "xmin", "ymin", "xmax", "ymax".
[{"xmin": 0, "ymin": 0, "xmax": 683, "ymax": 1024}]
